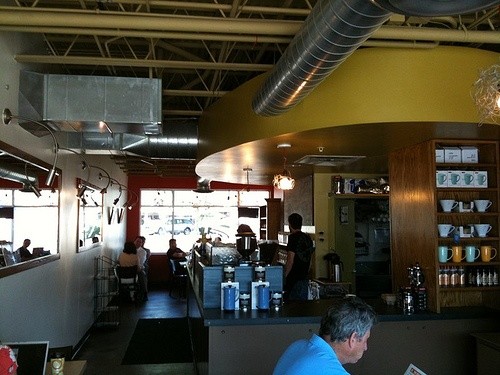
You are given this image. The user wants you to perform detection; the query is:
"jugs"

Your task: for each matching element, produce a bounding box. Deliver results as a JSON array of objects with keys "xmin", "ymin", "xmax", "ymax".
[
  {"xmin": 256, "ymin": 284, "xmax": 273, "ymax": 312},
  {"xmin": 223, "ymin": 285, "xmax": 241, "ymax": 312}
]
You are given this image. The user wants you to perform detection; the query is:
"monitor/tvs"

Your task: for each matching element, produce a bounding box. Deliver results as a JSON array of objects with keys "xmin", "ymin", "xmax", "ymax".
[{"xmin": 2, "ymin": 341, "xmax": 50, "ymax": 375}]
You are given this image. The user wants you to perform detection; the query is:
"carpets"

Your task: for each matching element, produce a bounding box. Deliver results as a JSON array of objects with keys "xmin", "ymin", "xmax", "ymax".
[{"xmin": 121, "ymin": 318, "xmax": 208, "ymax": 365}]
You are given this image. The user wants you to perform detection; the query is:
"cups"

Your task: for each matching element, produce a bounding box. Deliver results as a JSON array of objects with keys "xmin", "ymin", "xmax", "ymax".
[
  {"xmin": 452, "ymin": 246, "xmax": 469, "ymax": 263},
  {"xmin": 473, "ymin": 200, "xmax": 492, "ymax": 212},
  {"xmin": 438, "ymin": 173, "xmax": 447, "ymax": 184},
  {"xmin": 481, "ymin": 246, "xmax": 497, "ymax": 263},
  {"xmin": 438, "ymin": 246, "xmax": 453, "ymax": 264},
  {"xmin": 50, "ymin": 352, "xmax": 65, "ymax": 375},
  {"xmin": 451, "ymin": 174, "xmax": 460, "ymax": 184},
  {"xmin": 464, "ymin": 174, "xmax": 473, "ymax": 185},
  {"xmin": 438, "ymin": 224, "xmax": 455, "ymax": 237},
  {"xmin": 465, "ymin": 246, "xmax": 481, "ymax": 262},
  {"xmin": 474, "ymin": 224, "xmax": 492, "ymax": 238},
  {"xmin": 477, "ymin": 174, "xmax": 486, "ymax": 185},
  {"xmin": 440, "ymin": 199, "xmax": 459, "ymax": 213}
]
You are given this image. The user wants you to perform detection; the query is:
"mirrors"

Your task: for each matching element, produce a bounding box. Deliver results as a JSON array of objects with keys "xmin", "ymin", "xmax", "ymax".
[
  {"xmin": 0, "ymin": 140, "xmax": 61, "ymax": 278},
  {"xmin": 77, "ymin": 177, "xmax": 104, "ymax": 253}
]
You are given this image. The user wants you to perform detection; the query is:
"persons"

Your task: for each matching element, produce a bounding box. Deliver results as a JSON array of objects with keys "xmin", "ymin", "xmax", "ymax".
[
  {"xmin": 119, "ymin": 241, "xmax": 138, "ymax": 301},
  {"xmin": 17, "ymin": 238, "xmax": 31, "ymax": 262},
  {"xmin": 80, "ymin": 240, "xmax": 83, "ymax": 247},
  {"xmin": 272, "ymin": 296, "xmax": 377, "ymax": 375},
  {"xmin": 92, "ymin": 237, "xmax": 98, "ymax": 243},
  {"xmin": 167, "ymin": 238, "xmax": 189, "ymax": 276},
  {"xmin": 283, "ymin": 213, "xmax": 313, "ymax": 300},
  {"xmin": 134, "ymin": 236, "xmax": 151, "ymax": 301}
]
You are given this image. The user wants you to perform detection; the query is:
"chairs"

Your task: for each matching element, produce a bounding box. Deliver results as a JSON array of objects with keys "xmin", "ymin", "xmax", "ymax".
[{"xmin": 113, "ymin": 249, "xmax": 188, "ymax": 305}]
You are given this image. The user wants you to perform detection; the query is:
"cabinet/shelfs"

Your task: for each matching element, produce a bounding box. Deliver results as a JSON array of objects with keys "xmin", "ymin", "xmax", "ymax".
[
  {"xmin": 257, "ymin": 198, "xmax": 281, "ymax": 241},
  {"xmin": 388, "ymin": 138, "xmax": 500, "ymax": 315},
  {"xmin": 95, "ymin": 254, "xmax": 121, "ymax": 332}
]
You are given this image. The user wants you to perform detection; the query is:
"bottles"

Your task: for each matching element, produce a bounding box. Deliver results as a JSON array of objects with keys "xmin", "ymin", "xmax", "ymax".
[
  {"xmin": 381, "ymin": 264, "xmax": 427, "ymax": 316},
  {"xmin": 439, "ymin": 266, "xmax": 500, "ymax": 287},
  {"xmin": 334, "ymin": 175, "xmax": 343, "ymax": 195},
  {"xmin": 350, "ymin": 179, "xmax": 355, "ymax": 192}
]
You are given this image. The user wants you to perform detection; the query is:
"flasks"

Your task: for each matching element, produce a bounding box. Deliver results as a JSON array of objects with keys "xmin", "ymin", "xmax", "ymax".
[{"xmin": 333, "ymin": 263, "xmax": 341, "ymax": 282}]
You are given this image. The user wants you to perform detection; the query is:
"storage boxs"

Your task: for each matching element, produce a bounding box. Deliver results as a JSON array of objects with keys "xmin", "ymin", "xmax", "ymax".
[{"xmin": 434, "ymin": 146, "xmax": 488, "ymax": 188}]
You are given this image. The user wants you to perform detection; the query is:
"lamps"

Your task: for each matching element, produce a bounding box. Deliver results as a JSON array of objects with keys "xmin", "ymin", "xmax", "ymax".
[
  {"xmin": 272, "ymin": 157, "xmax": 296, "ymax": 190},
  {"xmin": 90, "ymin": 195, "xmax": 99, "ymax": 207},
  {"xmin": 24, "ymin": 162, "xmax": 41, "ymax": 198},
  {"xmin": 3, "ymin": 108, "xmax": 138, "ymax": 209},
  {"xmin": 81, "ymin": 191, "xmax": 87, "ymax": 206}
]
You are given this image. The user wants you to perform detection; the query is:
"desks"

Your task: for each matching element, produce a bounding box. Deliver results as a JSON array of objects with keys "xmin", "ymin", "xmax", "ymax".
[
  {"xmin": 470, "ymin": 332, "xmax": 500, "ymax": 375},
  {"xmin": 313, "ymin": 278, "xmax": 352, "ymax": 299}
]
